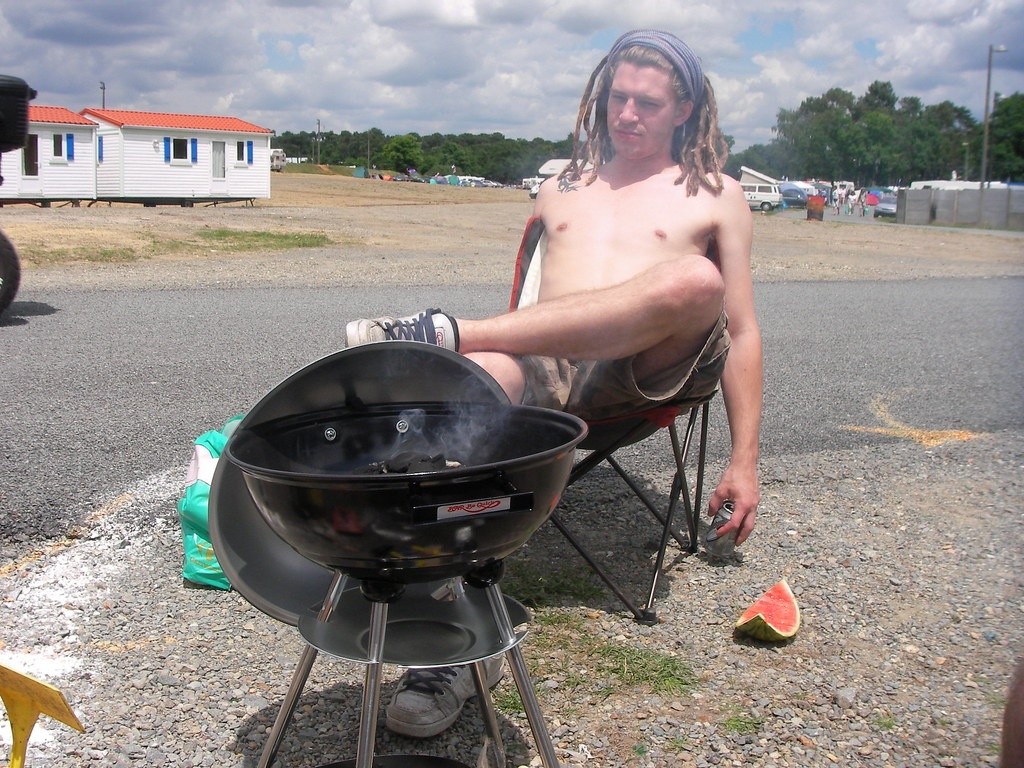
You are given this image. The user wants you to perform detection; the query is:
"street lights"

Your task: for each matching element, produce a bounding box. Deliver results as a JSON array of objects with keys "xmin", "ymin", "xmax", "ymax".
[
  {"xmin": 978, "ymin": 44, "xmax": 1009, "ymax": 190},
  {"xmin": 961, "ymin": 141, "xmax": 970, "ymax": 181}
]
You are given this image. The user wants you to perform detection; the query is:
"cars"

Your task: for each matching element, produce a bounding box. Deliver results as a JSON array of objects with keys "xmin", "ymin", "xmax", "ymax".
[
  {"xmin": 874, "ymin": 196, "xmax": 898, "ymax": 218},
  {"xmin": 392, "ymin": 175, "xmax": 505, "ymax": 188}
]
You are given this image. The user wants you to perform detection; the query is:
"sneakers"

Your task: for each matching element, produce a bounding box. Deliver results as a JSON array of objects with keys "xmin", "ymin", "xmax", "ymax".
[
  {"xmin": 386, "ymin": 652, "xmax": 504, "ymax": 736},
  {"xmin": 345, "ymin": 308, "xmax": 459, "ymax": 354}
]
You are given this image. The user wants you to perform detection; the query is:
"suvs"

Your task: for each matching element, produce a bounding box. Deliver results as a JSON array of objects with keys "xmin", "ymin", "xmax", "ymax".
[{"xmin": 739, "ymin": 183, "xmax": 783, "ymax": 212}]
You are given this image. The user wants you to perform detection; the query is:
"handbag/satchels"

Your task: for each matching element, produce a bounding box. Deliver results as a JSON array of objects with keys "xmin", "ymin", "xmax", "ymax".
[{"xmin": 179, "ymin": 415, "xmax": 246, "ymax": 593}]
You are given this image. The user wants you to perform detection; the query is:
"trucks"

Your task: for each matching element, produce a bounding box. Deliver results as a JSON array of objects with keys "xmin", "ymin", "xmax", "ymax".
[
  {"xmin": 270, "ymin": 148, "xmax": 287, "ymax": 172},
  {"xmin": 529, "ymin": 157, "xmax": 593, "ymax": 199}
]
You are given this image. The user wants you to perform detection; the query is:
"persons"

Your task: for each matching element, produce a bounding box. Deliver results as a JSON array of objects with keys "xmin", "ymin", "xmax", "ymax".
[
  {"xmin": 816, "ymin": 184, "xmax": 867, "ymax": 217},
  {"xmin": 344, "ymin": 28, "xmax": 765, "ymax": 739}
]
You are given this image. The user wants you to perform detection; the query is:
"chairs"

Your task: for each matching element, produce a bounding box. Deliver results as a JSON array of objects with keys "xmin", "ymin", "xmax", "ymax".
[{"xmin": 509, "ymin": 215, "xmax": 721, "ymax": 628}]
